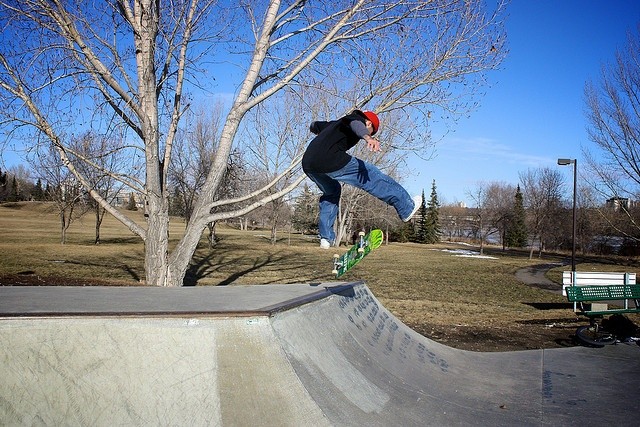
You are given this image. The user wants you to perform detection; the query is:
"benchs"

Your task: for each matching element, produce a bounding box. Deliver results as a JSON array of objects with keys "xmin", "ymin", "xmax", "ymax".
[{"xmin": 565, "ymin": 284, "xmax": 640, "ymax": 346}]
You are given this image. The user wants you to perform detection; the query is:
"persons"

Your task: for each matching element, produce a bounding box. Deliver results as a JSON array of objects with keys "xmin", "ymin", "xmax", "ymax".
[{"xmin": 302, "ymin": 110, "xmax": 423, "ymax": 250}]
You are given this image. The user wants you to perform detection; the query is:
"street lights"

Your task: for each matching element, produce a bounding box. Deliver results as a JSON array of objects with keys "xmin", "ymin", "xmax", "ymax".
[{"xmin": 558, "ymin": 157, "xmax": 578, "ymax": 273}]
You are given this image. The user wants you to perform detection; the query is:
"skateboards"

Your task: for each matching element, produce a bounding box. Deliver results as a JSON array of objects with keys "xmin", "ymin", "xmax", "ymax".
[{"xmin": 332, "ymin": 229, "xmax": 383, "ymax": 279}]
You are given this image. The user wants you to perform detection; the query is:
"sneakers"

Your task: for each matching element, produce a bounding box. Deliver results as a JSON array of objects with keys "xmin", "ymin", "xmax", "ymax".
[
  {"xmin": 402, "ymin": 195, "xmax": 422, "ymax": 222},
  {"xmin": 320, "ymin": 238, "xmax": 330, "ymax": 249}
]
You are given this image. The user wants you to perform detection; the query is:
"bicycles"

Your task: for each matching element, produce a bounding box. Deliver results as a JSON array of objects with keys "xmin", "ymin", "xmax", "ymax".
[{"xmin": 579, "ymin": 316, "xmax": 640, "ymax": 348}]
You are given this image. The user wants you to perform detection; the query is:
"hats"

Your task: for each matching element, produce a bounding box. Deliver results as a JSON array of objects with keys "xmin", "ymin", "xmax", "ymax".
[{"xmin": 353, "ymin": 109, "xmax": 379, "ymax": 136}]
